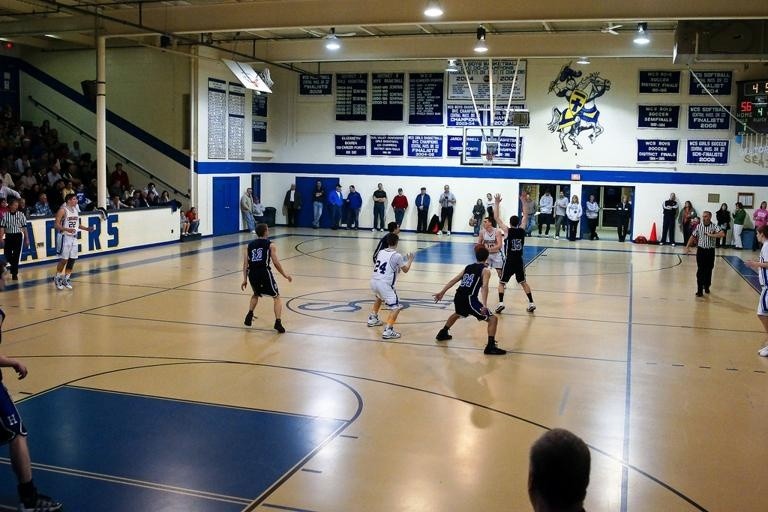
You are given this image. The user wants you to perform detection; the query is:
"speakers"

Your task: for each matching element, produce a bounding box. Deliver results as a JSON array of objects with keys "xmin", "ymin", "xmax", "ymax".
[{"xmin": 80, "ymin": 79, "xmax": 97, "ymax": 99}]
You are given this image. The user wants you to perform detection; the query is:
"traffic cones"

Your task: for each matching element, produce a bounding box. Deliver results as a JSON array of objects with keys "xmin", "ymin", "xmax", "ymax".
[{"xmin": 648, "ymin": 222, "xmax": 659, "ymax": 245}]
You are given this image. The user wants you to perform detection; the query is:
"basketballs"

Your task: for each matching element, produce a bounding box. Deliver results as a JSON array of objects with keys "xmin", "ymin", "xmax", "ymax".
[{"xmin": 474, "ymin": 244, "xmax": 485, "ymax": 252}]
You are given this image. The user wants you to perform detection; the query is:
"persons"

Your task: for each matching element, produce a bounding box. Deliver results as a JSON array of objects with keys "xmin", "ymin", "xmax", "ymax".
[
  {"xmin": 284, "ymin": 184, "xmax": 303, "ymax": 228},
  {"xmin": 366, "ymin": 233, "xmax": 415, "ymax": 340},
  {"xmin": 527, "ymin": 427, "xmax": 592, "ymax": 511},
  {"xmin": 240, "ymin": 188, "xmax": 257, "ymax": 234},
  {"xmin": 326, "ymin": 183, "xmax": 344, "ymax": 230},
  {"xmin": 415, "ymin": 187, "xmax": 430, "ymax": 233},
  {"xmin": 390, "ymin": 187, "xmax": 408, "ymax": 229},
  {"xmin": 310, "ymin": 180, "xmax": 328, "ymax": 229},
  {"xmin": 472, "ymin": 188, "xmax": 768, "ymax": 247},
  {"xmin": 371, "ymin": 183, "xmax": 387, "ymax": 232},
  {"xmin": 436, "ymin": 184, "xmax": 456, "ymax": 236},
  {"xmin": 477, "ymin": 215, "xmax": 508, "ymax": 289},
  {"xmin": 0, "ymin": 97, "xmax": 200, "ymax": 292},
  {"xmin": 492, "ymin": 215, "xmax": 536, "ymax": 315},
  {"xmin": 684, "ymin": 210, "xmax": 725, "ymax": 298},
  {"xmin": 345, "ymin": 184, "xmax": 362, "ymax": 230},
  {"xmin": 0, "ymin": 254, "xmax": 65, "ymax": 511},
  {"xmin": 432, "ymin": 246, "xmax": 507, "ymax": 355},
  {"xmin": 240, "ymin": 222, "xmax": 292, "ymax": 333},
  {"xmin": 372, "ymin": 222, "xmax": 400, "ymax": 265},
  {"xmin": 251, "ymin": 196, "xmax": 267, "ymax": 224},
  {"xmin": 743, "ymin": 224, "xmax": 768, "ymax": 358}
]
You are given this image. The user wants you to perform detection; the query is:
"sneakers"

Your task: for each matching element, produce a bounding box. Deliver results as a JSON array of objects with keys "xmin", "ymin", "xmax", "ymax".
[
  {"xmin": 528, "ymin": 234, "xmax": 531, "ymax": 237},
  {"xmin": 660, "ymin": 242, "xmax": 663, "ymax": 245},
  {"xmin": 758, "ymin": 346, "xmax": 768, "ymax": 356},
  {"xmin": 244, "ymin": 303, "xmax": 536, "ymax": 355},
  {"xmin": 671, "ymin": 243, "xmax": 676, "ymax": 246},
  {"xmin": 696, "ymin": 286, "xmax": 710, "ymax": 296},
  {"xmin": 53, "ymin": 276, "xmax": 72, "ymax": 290},
  {"xmin": 436, "ymin": 230, "xmax": 451, "ymax": 236},
  {"xmin": 181, "ymin": 231, "xmax": 202, "ymax": 237},
  {"xmin": 371, "ymin": 228, "xmax": 384, "ymax": 232},
  {"xmin": 12, "ymin": 275, "xmax": 17, "ymax": 280},
  {"xmin": 346, "ymin": 227, "xmax": 358, "ymax": 230},
  {"xmin": 473, "ymin": 234, "xmax": 478, "ymax": 237},
  {"xmin": 16, "ymin": 494, "xmax": 63, "ymax": 512},
  {"xmin": 250, "ymin": 230, "xmax": 256, "ymax": 234}
]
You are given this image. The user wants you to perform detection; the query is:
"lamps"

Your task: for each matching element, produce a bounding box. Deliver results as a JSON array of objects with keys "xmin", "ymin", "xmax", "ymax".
[
  {"xmin": 424, "ymin": 0, "xmax": 443, "ymax": 17},
  {"xmin": 474, "ymin": 24, "xmax": 489, "ymax": 52},
  {"xmin": 577, "ymin": 58, "xmax": 590, "ymax": 64},
  {"xmin": 633, "ymin": 23, "xmax": 650, "ymax": 45},
  {"xmin": 324, "ymin": 28, "xmax": 341, "ymax": 50},
  {"xmin": 445, "ymin": 59, "xmax": 458, "ymax": 71}
]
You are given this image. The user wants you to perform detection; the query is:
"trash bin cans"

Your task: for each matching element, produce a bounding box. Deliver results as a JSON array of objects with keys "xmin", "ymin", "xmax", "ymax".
[
  {"xmin": 260, "ymin": 207, "xmax": 276, "ymax": 227},
  {"xmin": 741, "ymin": 228, "xmax": 755, "ymax": 250}
]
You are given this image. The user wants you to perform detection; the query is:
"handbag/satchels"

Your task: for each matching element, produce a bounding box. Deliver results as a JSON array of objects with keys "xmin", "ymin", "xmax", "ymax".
[{"xmin": 469, "ymin": 218, "xmax": 478, "ymax": 226}]
